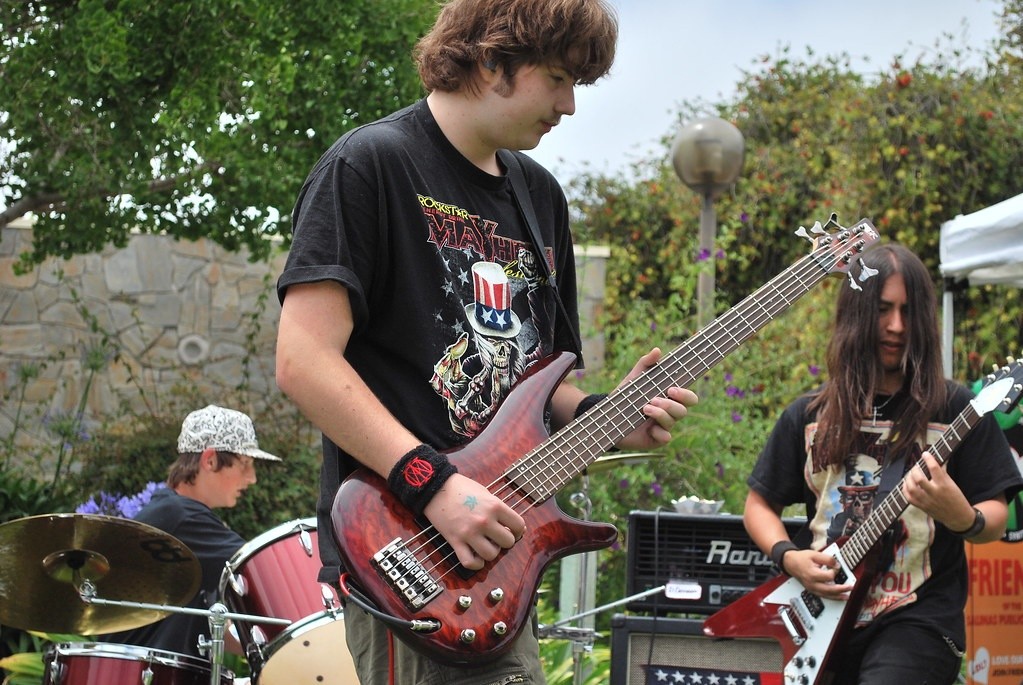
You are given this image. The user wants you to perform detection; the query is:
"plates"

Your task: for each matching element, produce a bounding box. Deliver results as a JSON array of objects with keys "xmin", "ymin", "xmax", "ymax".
[{"xmin": 671, "ymin": 499, "xmax": 726, "ymax": 514}]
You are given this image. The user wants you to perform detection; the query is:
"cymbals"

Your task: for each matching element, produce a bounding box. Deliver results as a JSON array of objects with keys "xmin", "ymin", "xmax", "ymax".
[{"xmin": 0, "ymin": 511, "xmax": 202, "ymax": 635}]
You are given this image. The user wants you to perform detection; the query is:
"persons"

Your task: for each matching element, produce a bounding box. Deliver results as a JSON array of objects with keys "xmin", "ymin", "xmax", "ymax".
[
  {"xmin": 267, "ymin": 0, "xmax": 698, "ymax": 685},
  {"xmin": 90, "ymin": 404, "xmax": 285, "ymax": 685},
  {"xmin": 744, "ymin": 241, "xmax": 1023, "ymax": 685}
]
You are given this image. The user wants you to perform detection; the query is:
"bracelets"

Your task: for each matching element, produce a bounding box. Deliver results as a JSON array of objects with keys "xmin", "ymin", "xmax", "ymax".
[
  {"xmin": 378, "ymin": 441, "xmax": 459, "ymax": 513},
  {"xmin": 960, "ymin": 506, "xmax": 986, "ymax": 541},
  {"xmin": 574, "ymin": 390, "xmax": 619, "ymax": 453},
  {"xmin": 767, "ymin": 540, "xmax": 799, "ymax": 578}
]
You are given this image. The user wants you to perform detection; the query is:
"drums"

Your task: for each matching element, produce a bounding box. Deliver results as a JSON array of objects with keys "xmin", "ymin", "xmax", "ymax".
[{"xmin": 42, "ymin": 516, "xmax": 358, "ymax": 685}]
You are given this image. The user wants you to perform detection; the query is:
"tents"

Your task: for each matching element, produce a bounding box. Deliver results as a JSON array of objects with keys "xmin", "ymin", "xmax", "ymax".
[{"xmin": 937, "ymin": 193, "xmax": 1023, "ymax": 379}]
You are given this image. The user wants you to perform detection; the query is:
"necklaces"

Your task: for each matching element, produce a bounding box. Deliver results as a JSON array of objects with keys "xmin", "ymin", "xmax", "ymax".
[{"xmin": 856, "ymin": 373, "xmax": 901, "ymax": 427}]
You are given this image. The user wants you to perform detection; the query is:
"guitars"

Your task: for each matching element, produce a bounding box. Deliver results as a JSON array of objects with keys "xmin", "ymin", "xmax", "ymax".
[
  {"xmin": 700, "ymin": 357, "xmax": 1023, "ymax": 685},
  {"xmin": 326, "ymin": 212, "xmax": 880, "ymax": 667}
]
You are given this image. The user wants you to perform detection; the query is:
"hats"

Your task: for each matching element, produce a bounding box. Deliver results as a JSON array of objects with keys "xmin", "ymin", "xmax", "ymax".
[{"xmin": 176, "ymin": 404, "xmax": 283, "ymax": 463}]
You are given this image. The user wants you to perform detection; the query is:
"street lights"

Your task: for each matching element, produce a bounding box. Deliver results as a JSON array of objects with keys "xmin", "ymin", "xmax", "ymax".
[{"xmin": 671, "ymin": 114, "xmax": 745, "ymax": 328}]
